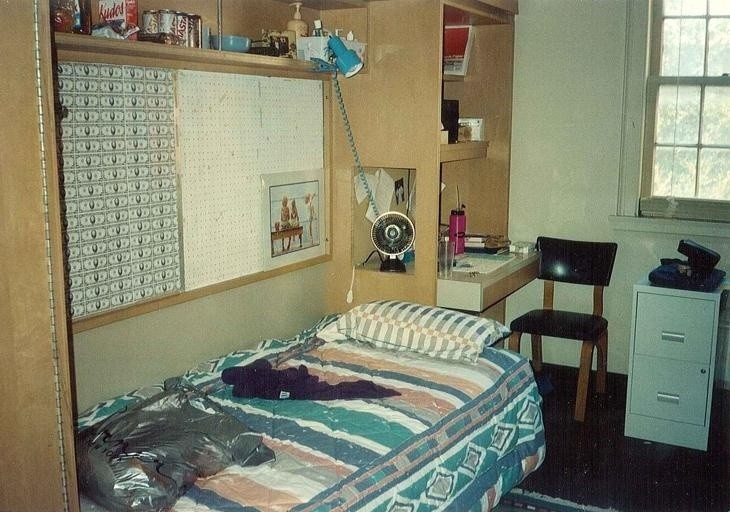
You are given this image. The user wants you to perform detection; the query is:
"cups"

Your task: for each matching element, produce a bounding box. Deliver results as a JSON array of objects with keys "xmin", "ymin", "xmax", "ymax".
[{"xmin": 439, "ymin": 242, "xmax": 455, "ymax": 280}]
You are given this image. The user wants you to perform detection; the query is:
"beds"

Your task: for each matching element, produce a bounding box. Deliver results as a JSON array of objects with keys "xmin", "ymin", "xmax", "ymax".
[{"xmin": 76, "ymin": 310, "xmax": 545, "ymax": 512}]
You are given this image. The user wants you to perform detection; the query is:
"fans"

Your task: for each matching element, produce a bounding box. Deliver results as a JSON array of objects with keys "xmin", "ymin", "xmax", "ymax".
[{"xmin": 370, "ymin": 212, "xmax": 415, "ymax": 271}]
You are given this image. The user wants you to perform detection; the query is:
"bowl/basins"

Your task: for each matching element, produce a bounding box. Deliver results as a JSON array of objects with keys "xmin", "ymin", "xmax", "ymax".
[{"xmin": 211, "ymin": 35, "xmax": 251, "ymax": 53}]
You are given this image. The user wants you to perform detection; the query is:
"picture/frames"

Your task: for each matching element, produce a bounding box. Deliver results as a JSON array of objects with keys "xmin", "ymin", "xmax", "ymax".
[{"xmin": 259, "ymin": 168, "xmax": 327, "ymax": 271}]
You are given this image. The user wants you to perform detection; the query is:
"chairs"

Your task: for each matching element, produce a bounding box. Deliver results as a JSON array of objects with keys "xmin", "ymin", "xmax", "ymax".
[{"xmin": 508, "ymin": 237, "xmax": 617, "ymax": 422}]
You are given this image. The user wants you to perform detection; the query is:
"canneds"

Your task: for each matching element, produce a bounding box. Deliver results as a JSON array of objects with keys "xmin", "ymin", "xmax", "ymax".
[
  {"xmin": 187, "ymin": 13, "xmax": 203, "ymax": 49},
  {"xmin": 175, "ymin": 11, "xmax": 189, "ymax": 47},
  {"xmin": 141, "ymin": 9, "xmax": 159, "ymax": 33},
  {"xmin": 157, "ymin": 8, "xmax": 177, "ymax": 35}
]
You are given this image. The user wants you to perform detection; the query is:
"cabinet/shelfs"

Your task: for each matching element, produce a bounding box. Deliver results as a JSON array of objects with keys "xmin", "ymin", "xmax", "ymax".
[
  {"xmin": 623, "ymin": 279, "xmax": 724, "ymax": 452},
  {"xmin": 49, "ymin": 0, "xmax": 370, "ymax": 76},
  {"xmin": 330, "ymin": 0, "xmax": 541, "ymax": 351}
]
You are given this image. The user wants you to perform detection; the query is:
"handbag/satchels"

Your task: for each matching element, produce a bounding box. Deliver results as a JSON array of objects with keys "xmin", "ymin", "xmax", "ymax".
[{"xmin": 76, "ymin": 375, "xmax": 275, "ymax": 512}]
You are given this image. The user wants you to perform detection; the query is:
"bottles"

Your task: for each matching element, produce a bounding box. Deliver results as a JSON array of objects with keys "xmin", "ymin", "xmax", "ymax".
[{"xmin": 449, "ymin": 208, "xmax": 466, "ymax": 254}]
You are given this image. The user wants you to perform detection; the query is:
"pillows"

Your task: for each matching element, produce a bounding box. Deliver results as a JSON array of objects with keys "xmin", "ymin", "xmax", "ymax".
[{"xmin": 317, "ymin": 297, "xmax": 514, "ymax": 365}]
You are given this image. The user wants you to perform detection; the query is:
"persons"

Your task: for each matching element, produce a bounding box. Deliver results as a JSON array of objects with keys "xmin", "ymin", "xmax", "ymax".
[
  {"xmin": 291, "ymin": 200, "xmax": 302, "ymax": 242},
  {"xmin": 281, "ymin": 197, "xmax": 292, "ymax": 251},
  {"xmin": 304, "ymin": 194, "xmax": 316, "ymax": 245}
]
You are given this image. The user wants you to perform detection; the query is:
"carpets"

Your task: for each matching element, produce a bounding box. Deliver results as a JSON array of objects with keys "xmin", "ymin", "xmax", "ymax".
[{"xmin": 491, "ymin": 488, "xmax": 619, "ymax": 512}]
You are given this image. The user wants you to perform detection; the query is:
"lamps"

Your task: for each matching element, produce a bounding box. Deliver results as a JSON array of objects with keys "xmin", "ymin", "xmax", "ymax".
[{"xmin": 327, "ymin": 34, "xmax": 364, "ymax": 78}]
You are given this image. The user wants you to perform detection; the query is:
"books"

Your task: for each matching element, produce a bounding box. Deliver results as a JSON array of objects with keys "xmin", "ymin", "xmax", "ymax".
[{"xmin": 444, "ymin": 228, "xmax": 536, "ymax": 256}]
